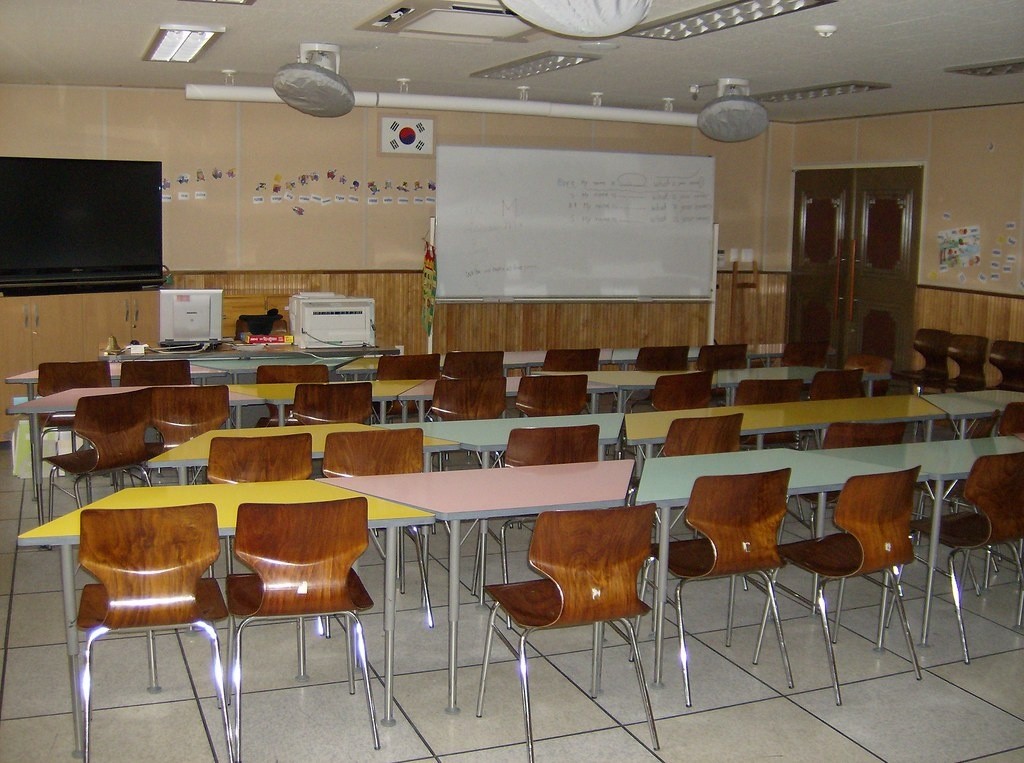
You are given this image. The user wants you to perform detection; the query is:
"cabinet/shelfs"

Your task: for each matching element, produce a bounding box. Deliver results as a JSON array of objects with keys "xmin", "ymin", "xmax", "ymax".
[
  {"xmin": 222, "ymin": 288, "xmax": 290, "ymax": 340},
  {"xmin": 84, "ymin": 292, "xmax": 163, "ymax": 359},
  {"xmin": 0, "ymin": 294, "xmax": 85, "ymax": 442}
]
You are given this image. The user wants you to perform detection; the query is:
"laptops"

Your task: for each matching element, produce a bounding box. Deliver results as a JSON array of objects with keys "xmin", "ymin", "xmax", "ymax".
[{"xmin": 160, "ymin": 290, "xmax": 223, "ymax": 349}]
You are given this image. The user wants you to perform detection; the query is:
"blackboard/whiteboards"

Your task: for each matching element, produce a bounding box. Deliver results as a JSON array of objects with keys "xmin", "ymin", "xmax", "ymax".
[{"xmin": 434, "ymin": 145, "xmax": 717, "ymax": 302}]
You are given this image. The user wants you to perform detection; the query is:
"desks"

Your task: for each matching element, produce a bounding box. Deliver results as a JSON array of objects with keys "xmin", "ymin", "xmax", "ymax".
[
  {"xmin": 16, "ymin": 479, "xmax": 435, "ymax": 762},
  {"xmin": 3, "ymin": 359, "xmax": 228, "ymax": 501},
  {"xmin": 6, "ymin": 385, "xmax": 267, "ymax": 550},
  {"xmin": 920, "ymin": 389, "xmax": 1024, "ymax": 440},
  {"xmin": 453, "ymin": 348, "xmax": 613, "ymax": 376},
  {"xmin": 146, "ymin": 422, "xmax": 459, "ymax": 612},
  {"xmin": 401, "ymin": 371, "xmax": 617, "ymax": 422},
  {"xmin": 806, "ymin": 435, "xmax": 1022, "ymax": 647},
  {"xmin": 97, "ymin": 329, "xmax": 407, "ymax": 371},
  {"xmin": 315, "ymin": 457, "xmax": 635, "ymax": 715},
  {"xmin": 612, "ymin": 346, "xmax": 702, "ymax": 369},
  {"xmin": 337, "ymin": 355, "xmax": 446, "ymax": 381},
  {"xmin": 636, "ymin": 446, "xmax": 927, "ymax": 689},
  {"xmin": 372, "ymin": 413, "xmax": 625, "ymax": 609},
  {"xmin": 185, "ymin": 356, "xmax": 358, "ymax": 384},
  {"xmin": 718, "ymin": 364, "xmax": 891, "ymax": 405},
  {"xmin": 625, "ymin": 394, "xmax": 947, "ymax": 557},
  {"xmin": 746, "ymin": 343, "xmax": 837, "ymax": 368},
  {"xmin": 227, "ymin": 379, "xmax": 428, "ymax": 426},
  {"xmin": 534, "ymin": 368, "xmax": 719, "ymax": 458}
]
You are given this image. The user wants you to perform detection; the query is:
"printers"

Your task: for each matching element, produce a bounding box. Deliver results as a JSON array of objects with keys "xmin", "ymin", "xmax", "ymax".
[{"xmin": 287, "ymin": 295, "xmax": 376, "ymax": 349}]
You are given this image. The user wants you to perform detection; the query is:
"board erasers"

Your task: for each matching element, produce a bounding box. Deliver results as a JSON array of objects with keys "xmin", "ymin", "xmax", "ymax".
[
  {"xmin": 636, "ymin": 294, "xmax": 654, "ymax": 302},
  {"xmin": 482, "ymin": 297, "xmax": 515, "ymax": 302}
]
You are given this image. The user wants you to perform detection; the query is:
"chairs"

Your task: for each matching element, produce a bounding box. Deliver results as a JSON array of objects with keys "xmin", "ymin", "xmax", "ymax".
[
  {"xmin": 77, "ymin": 502, "xmax": 236, "ymax": 762},
  {"xmin": 227, "ymin": 496, "xmax": 380, "ymax": 762},
  {"xmin": 628, "ymin": 467, "xmax": 795, "ymax": 706},
  {"xmin": 884, "ymin": 450, "xmax": 1024, "ymax": 664},
  {"xmin": 35, "ymin": 324, "xmax": 1024, "ymax": 633},
  {"xmin": 474, "ymin": 502, "xmax": 662, "ymax": 761},
  {"xmin": 753, "ymin": 464, "xmax": 921, "ymax": 705}
]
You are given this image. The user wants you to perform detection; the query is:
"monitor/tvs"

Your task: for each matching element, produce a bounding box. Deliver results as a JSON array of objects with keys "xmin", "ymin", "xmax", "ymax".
[{"xmin": 0, "ymin": 156, "xmax": 163, "ymax": 285}]
